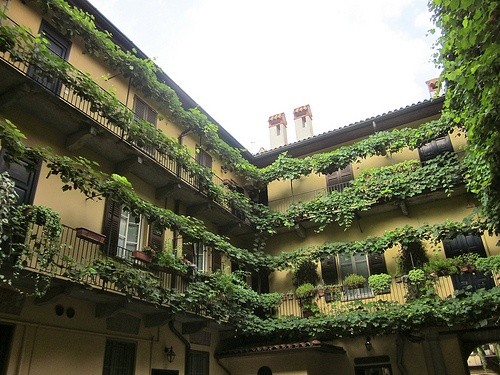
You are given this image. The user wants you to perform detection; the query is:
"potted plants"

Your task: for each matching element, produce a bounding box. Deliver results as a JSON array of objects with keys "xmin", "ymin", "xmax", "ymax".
[
  {"xmin": 20, "ymin": 204, "xmax": 60, "ymax": 252},
  {"xmin": 427, "ymin": 256, "xmax": 458, "ymax": 277},
  {"xmin": 342, "ymin": 274, "xmax": 366, "ymax": 289}
]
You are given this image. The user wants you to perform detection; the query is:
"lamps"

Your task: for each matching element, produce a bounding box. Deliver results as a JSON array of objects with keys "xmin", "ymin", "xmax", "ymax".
[
  {"xmin": 365, "ymin": 341, "xmax": 373, "ymax": 351},
  {"xmin": 164, "ymin": 346, "xmax": 175, "ymax": 363}
]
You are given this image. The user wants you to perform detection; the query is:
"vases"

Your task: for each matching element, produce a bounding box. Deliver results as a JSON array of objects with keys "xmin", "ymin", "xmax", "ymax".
[
  {"xmin": 77, "ymin": 227, "xmax": 107, "ymax": 244},
  {"xmin": 133, "ymin": 250, "xmax": 154, "ymax": 263}
]
